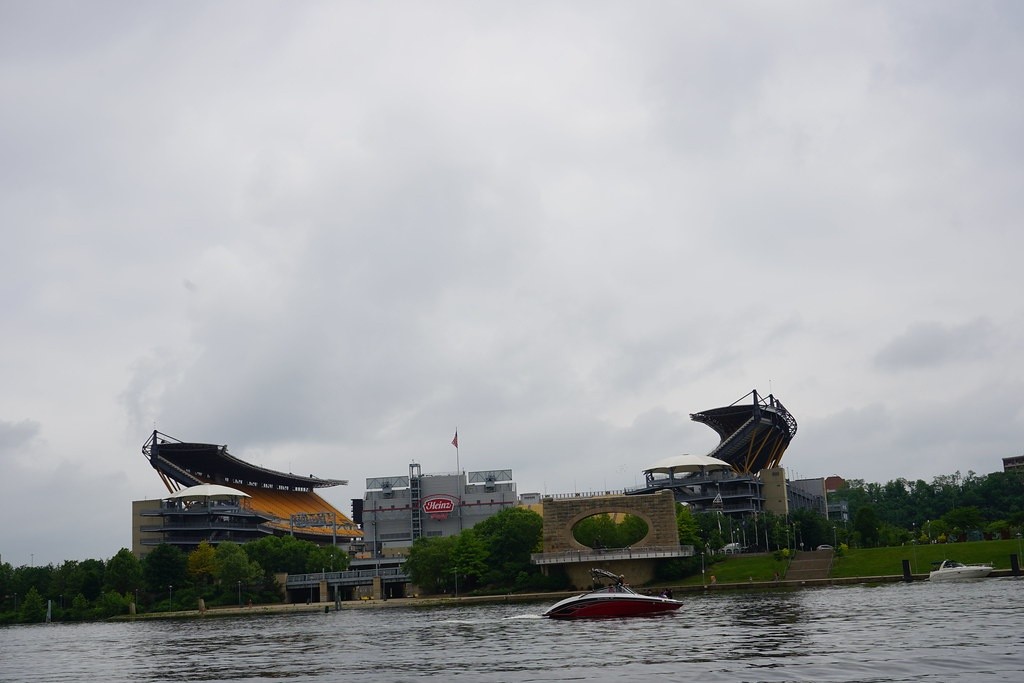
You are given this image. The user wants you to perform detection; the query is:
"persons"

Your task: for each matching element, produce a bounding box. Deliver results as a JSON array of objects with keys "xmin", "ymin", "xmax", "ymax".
[
  {"xmin": 642, "ymin": 588, "xmax": 673, "ymax": 599},
  {"xmin": 616, "ymin": 574, "xmax": 626, "ymax": 587},
  {"xmin": 608, "ymin": 583, "xmax": 614, "ymax": 593}
]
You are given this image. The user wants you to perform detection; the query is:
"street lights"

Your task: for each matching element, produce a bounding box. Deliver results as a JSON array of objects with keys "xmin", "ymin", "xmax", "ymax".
[
  {"xmin": 330, "ymin": 553, "xmax": 334, "ymax": 572},
  {"xmin": 135, "ymin": 588, "xmax": 139, "ymax": 614},
  {"xmin": 453, "ymin": 567, "xmax": 458, "ymax": 597},
  {"xmin": 14, "ymin": 592, "xmax": 17, "ymax": 612},
  {"xmin": 238, "ymin": 581, "xmax": 241, "ymax": 607},
  {"xmin": 309, "ymin": 577, "xmax": 313, "ymax": 605},
  {"xmin": 911, "ymin": 540, "xmax": 918, "ymax": 574},
  {"xmin": 101, "ymin": 591, "xmax": 105, "ymax": 601},
  {"xmin": 785, "ymin": 529, "xmax": 790, "ymax": 549},
  {"xmin": 701, "ymin": 551, "xmax": 705, "ymax": 586},
  {"xmin": 397, "ymin": 552, "xmax": 401, "ymax": 567},
  {"xmin": 169, "ymin": 585, "xmax": 173, "ymax": 612},
  {"xmin": 832, "ymin": 526, "xmax": 837, "ymax": 549},
  {"xmin": 59, "ymin": 594, "xmax": 63, "ymax": 609},
  {"xmin": 345, "ymin": 555, "xmax": 348, "ymax": 571},
  {"xmin": 1016, "ymin": 533, "xmax": 1023, "ymax": 570},
  {"xmin": 377, "ymin": 549, "xmax": 381, "ymax": 569}
]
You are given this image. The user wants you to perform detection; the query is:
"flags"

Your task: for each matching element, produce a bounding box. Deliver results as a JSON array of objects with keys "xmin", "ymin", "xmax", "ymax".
[{"xmin": 451, "ymin": 430, "xmax": 458, "ymax": 448}]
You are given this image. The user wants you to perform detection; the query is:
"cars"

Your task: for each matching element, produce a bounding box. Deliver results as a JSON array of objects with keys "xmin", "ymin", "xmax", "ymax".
[{"xmin": 816, "ymin": 544, "xmax": 834, "ymax": 551}]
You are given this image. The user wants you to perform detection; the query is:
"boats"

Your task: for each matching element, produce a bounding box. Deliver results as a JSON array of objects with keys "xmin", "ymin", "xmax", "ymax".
[
  {"xmin": 924, "ymin": 559, "xmax": 997, "ymax": 582},
  {"xmin": 541, "ymin": 568, "xmax": 685, "ymax": 620}
]
